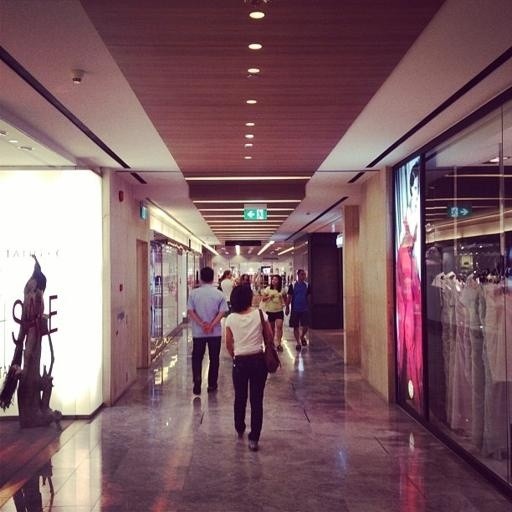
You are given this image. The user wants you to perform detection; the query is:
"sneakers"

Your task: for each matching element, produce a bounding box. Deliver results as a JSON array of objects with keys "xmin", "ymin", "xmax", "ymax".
[
  {"xmin": 301, "ymin": 336, "xmax": 307, "ymax": 346},
  {"xmin": 276, "ymin": 344, "xmax": 283, "ymax": 351},
  {"xmin": 296, "ymin": 344, "xmax": 301, "ymax": 350},
  {"xmin": 249, "ymin": 439, "xmax": 258, "ymax": 450},
  {"xmin": 236, "ymin": 424, "xmax": 246, "ymax": 434},
  {"xmin": 192, "ymin": 377, "xmax": 202, "ymax": 395},
  {"xmin": 207, "ymin": 383, "xmax": 218, "ymax": 392}
]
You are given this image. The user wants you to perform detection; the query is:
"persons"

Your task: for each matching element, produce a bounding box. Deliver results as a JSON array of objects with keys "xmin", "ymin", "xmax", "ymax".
[
  {"xmin": 186, "ymin": 266, "xmax": 229, "ymax": 394},
  {"xmin": 225, "ymin": 285, "xmax": 274, "ymax": 451},
  {"xmin": 217, "ymin": 269, "xmax": 312, "ymax": 353},
  {"xmin": 393, "ymin": 161, "xmax": 423, "ymax": 414}
]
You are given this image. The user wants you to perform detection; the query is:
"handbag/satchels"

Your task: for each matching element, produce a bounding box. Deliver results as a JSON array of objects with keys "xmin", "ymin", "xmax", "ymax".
[{"xmin": 265, "ymin": 345, "xmax": 281, "ymax": 374}]
